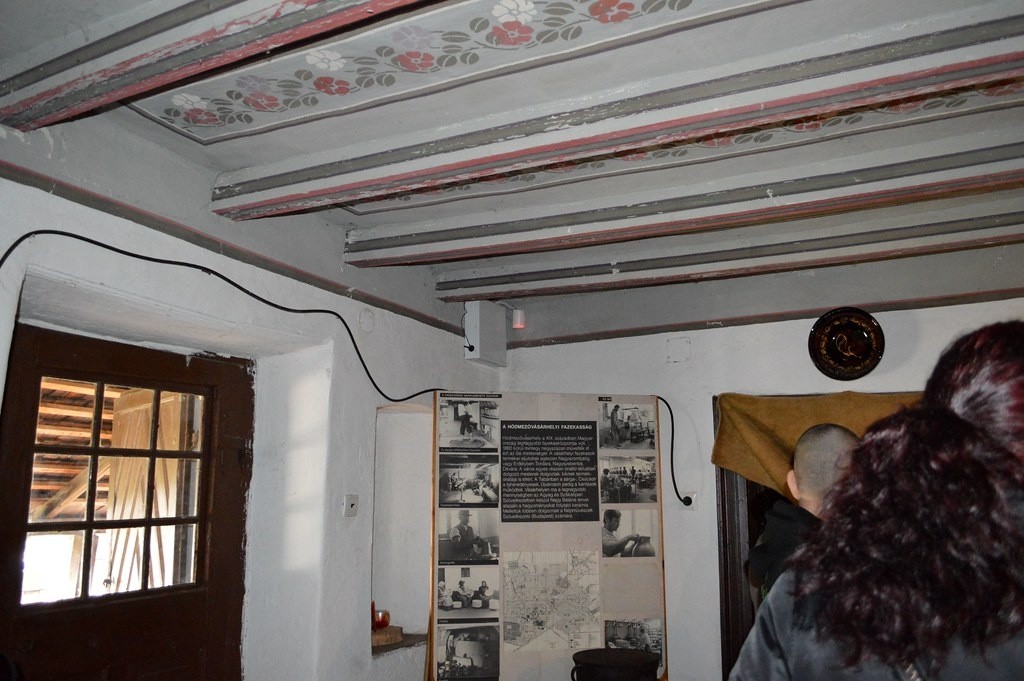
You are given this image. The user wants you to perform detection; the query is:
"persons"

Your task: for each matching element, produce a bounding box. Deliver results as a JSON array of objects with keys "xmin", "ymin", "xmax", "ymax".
[
  {"xmin": 637, "ymin": 628, "xmax": 652, "ymax": 651},
  {"xmin": 601, "ymin": 509, "xmax": 639, "ymax": 557},
  {"xmin": 610, "ymin": 404, "xmax": 635, "ymax": 447},
  {"xmin": 600, "ymin": 465, "xmax": 655, "ymax": 503},
  {"xmin": 727, "ymin": 317, "xmax": 1024, "ymax": 681},
  {"xmin": 438, "ymin": 580, "xmax": 492, "ymax": 610},
  {"xmin": 450, "ymin": 510, "xmax": 480, "ymax": 559}
]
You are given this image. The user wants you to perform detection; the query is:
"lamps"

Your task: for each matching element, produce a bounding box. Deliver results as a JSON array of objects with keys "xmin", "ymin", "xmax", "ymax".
[{"xmin": 464, "ymin": 300, "xmax": 525, "ymax": 369}]
